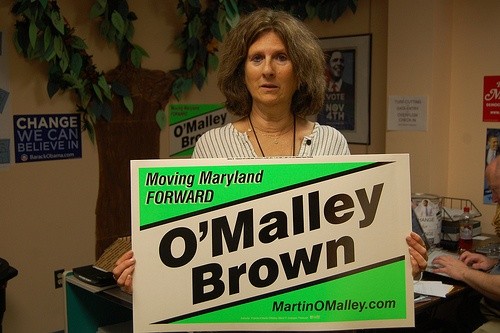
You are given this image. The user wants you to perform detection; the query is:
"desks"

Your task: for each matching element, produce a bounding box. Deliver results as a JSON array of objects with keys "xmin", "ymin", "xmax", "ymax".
[{"xmin": 63, "ymin": 231, "xmax": 500, "ymax": 333}]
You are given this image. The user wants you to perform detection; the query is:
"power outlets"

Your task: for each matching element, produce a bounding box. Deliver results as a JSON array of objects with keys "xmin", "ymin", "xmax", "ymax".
[{"xmin": 55, "ymin": 270, "xmax": 66, "ymax": 288}]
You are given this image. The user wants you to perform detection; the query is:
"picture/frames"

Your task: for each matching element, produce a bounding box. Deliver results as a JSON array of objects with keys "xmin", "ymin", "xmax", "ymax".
[{"xmin": 319, "ymin": 32, "xmax": 373, "ymax": 145}]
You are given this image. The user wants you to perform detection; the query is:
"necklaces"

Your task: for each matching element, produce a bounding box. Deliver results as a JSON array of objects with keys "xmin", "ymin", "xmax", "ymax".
[
  {"xmin": 248, "ymin": 112, "xmax": 295, "ymax": 157},
  {"xmin": 256, "ymin": 131, "xmax": 289, "ymax": 144}
]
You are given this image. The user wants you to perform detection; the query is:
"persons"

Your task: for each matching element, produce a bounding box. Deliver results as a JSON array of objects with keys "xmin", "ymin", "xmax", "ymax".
[
  {"xmin": 432, "ymin": 155, "xmax": 500, "ymax": 333},
  {"xmin": 485, "ymin": 136, "xmax": 500, "ymax": 166},
  {"xmin": 325, "ymin": 50, "xmax": 347, "ymax": 93},
  {"xmin": 112, "ymin": 9, "xmax": 427, "ymax": 292}
]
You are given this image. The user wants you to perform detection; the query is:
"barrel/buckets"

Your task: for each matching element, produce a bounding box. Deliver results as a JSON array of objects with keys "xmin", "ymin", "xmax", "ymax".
[{"xmin": 411, "ymin": 192, "xmax": 443, "ymax": 246}]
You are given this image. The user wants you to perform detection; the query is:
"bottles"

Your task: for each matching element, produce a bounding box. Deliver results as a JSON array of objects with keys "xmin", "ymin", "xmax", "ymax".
[{"xmin": 459, "ymin": 207, "xmax": 473, "ymax": 252}]
